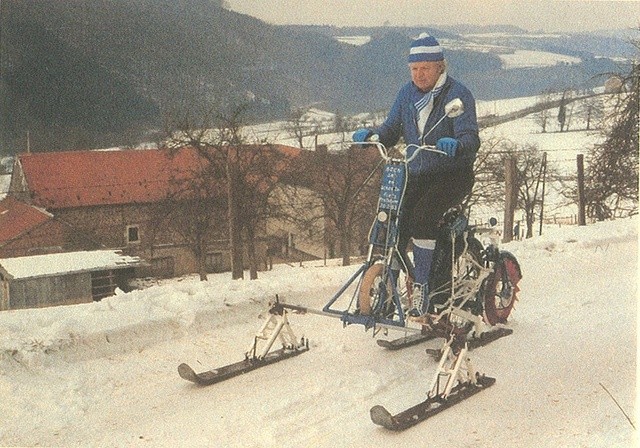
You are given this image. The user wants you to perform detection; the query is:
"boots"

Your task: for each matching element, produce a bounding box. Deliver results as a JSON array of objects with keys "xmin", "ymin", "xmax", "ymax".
[{"xmin": 408, "ymin": 282, "xmax": 430, "ymax": 322}]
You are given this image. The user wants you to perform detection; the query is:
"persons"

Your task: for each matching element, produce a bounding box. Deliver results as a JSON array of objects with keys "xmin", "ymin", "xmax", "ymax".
[
  {"xmin": 514, "ymin": 221, "xmax": 521, "ymax": 240},
  {"xmin": 352, "ymin": 32, "xmax": 480, "ymax": 318}
]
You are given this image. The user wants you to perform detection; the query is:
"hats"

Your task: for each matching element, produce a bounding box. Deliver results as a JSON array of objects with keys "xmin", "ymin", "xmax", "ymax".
[{"xmin": 408, "ymin": 37, "xmax": 444, "ymax": 62}]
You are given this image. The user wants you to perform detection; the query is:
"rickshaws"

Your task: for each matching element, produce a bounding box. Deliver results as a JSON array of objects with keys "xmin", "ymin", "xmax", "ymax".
[{"xmin": 170, "ymin": 136, "xmax": 522, "ymax": 432}]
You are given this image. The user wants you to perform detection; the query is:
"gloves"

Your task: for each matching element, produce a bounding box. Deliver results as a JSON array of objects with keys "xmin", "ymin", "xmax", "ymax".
[
  {"xmin": 436, "ymin": 137, "xmax": 464, "ymax": 159},
  {"xmin": 352, "ymin": 128, "xmax": 374, "ymax": 148}
]
